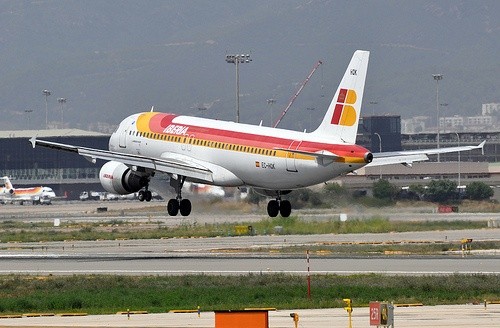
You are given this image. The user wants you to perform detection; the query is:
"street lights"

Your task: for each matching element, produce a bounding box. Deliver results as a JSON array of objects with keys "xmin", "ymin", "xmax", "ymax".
[
  {"xmin": 41, "ymin": 89, "xmax": 51, "ymax": 130},
  {"xmin": 432, "ymin": 74, "xmax": 444, "ymax": 162},
  {"xmin": 267, "ymin": 98, "xmax": 278, "ymax": 128},
  {"xmin": 198, "ymin": 107, "xmax": 207, "ymax": 118},
  {"xmin": 57, "ymin": 97, "xmax": 67, "ymax": 129},
  {"xmin": 307, "ymin": 107, "xmax": 316, "ymax": 133},
  {"xmin": 370, "ymin": 101, "xmax": 378, "ymax": 114},
  {"xmin": 225, "ymin": 53, "xmax": 252, "ymax": 124}
]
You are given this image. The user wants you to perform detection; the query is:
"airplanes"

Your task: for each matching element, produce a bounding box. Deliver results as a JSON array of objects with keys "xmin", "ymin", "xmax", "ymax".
[
  {"xmin": 0, "ymin": 176, "xmax": 56, "ymax": 205},
  {"xmin": 27, "ymin": 49, "xmax": 487, "ymax": 218},
  {"xmin": 173, "ymin": 174, "xmax": 225, "ymax": 203}
]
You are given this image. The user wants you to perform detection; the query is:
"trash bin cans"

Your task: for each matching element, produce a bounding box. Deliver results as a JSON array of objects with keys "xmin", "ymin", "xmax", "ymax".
[{"xmin": 369, "ymin": 300, "xmax": 394, "ymax": 328}]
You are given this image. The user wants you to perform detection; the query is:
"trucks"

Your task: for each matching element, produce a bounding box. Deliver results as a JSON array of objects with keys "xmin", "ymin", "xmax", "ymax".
[{"xmin": 80, "ymin": 191, "xmax": 107, "ymax": 201}]
